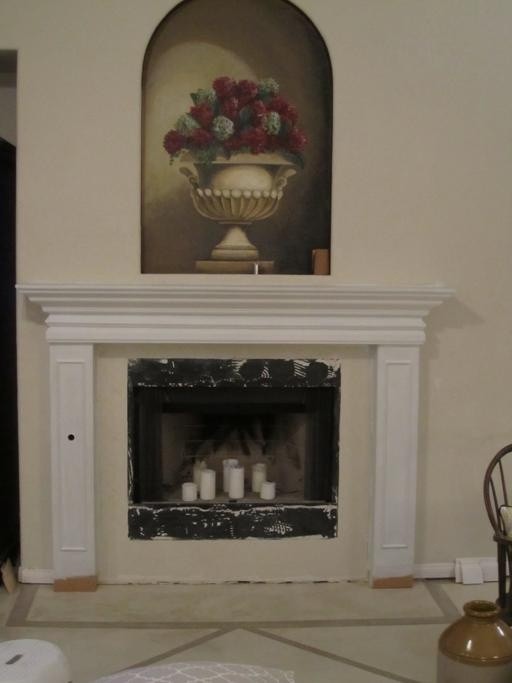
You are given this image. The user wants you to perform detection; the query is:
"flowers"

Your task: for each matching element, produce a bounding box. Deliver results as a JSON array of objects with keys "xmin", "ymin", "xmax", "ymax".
[{"xmin": 162, "ymin": 73, "xmax": 309, "ymax": 173}]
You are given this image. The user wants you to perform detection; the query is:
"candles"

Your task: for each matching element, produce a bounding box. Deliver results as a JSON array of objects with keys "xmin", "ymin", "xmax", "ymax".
[
  {"xmin": 200, "ymin": 468, "xmax": 216, "ymax": 500},
  {"xmin": 180, "ymin": 482, "xmax": 198, "ymax": 501},
  {"xmin": 222, "ymin": 458, "xmax": 239, "ymax": 493},
  {"xmin": 228, "ymin": 465, "xmax": 245, "ymax": 498},
  {"xmin": 259, "ymin": 480, "xmax": 276, "ymax": 500},
  {"xmin": 193, "ymin": 461, "xmax": 207, "ymax": 491},
  {"xmin": 251, "ymin": 462, "xmax": 267, "ymax": 493}
]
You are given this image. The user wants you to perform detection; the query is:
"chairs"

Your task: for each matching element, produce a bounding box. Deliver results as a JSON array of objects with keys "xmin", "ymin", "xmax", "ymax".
[{"xmin": 481, "ymin": 442, "xmax": 512, "ymax": 626}]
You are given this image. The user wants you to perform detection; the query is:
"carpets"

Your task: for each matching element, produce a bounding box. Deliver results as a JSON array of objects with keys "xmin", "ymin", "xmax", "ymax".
[{"xmin": 94, "ymin": 660, "xmax": 295, "ymax": 682}]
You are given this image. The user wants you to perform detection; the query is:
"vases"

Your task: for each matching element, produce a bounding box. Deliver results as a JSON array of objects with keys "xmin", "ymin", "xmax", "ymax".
[
  {"xmin": 180, "ymin": 152, "xmax": 297, "ymax": 260},
  {"xmin": 436, "ymin": 600, "xmax": 512, "ymax": 681}
]
